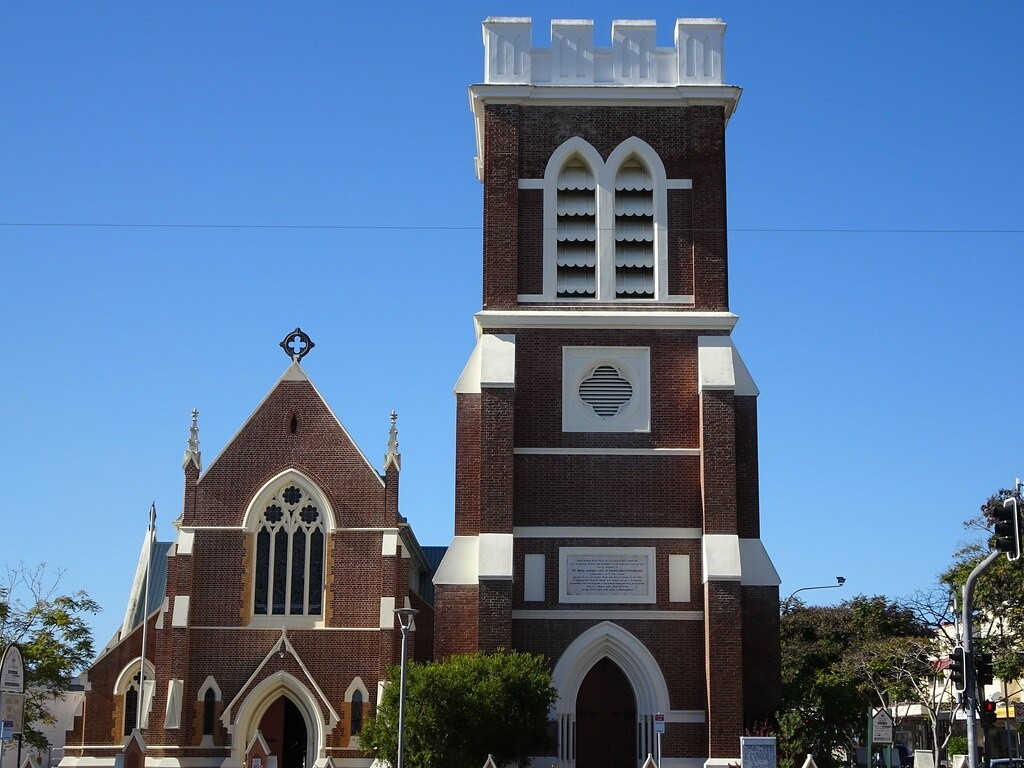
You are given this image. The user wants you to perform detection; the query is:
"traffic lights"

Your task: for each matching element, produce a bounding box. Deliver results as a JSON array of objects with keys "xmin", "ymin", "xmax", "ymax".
[
  {"xmin": 978, "ymin": 653, "xmax": 993, "ymax": 685},
  {"xmin": 993, "ymin": 497, "xmax": 1020, "ymax": 561},
  {"xmin": 949, "ymin": 646, "xmax": 966, "ymax": 693},
  {"xmin": 982, "ymin": 701, "xmax": 997, "ymax": 723}
]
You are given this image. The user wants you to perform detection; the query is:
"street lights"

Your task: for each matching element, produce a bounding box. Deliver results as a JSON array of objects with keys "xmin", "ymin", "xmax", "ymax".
[
  {"xmin": 393, "ymin": 608, "xmax": 421, "ymax": 768},
  {"xmin": 786, "ymin": 577, "xmax": 846, "ymax": 613}
]
